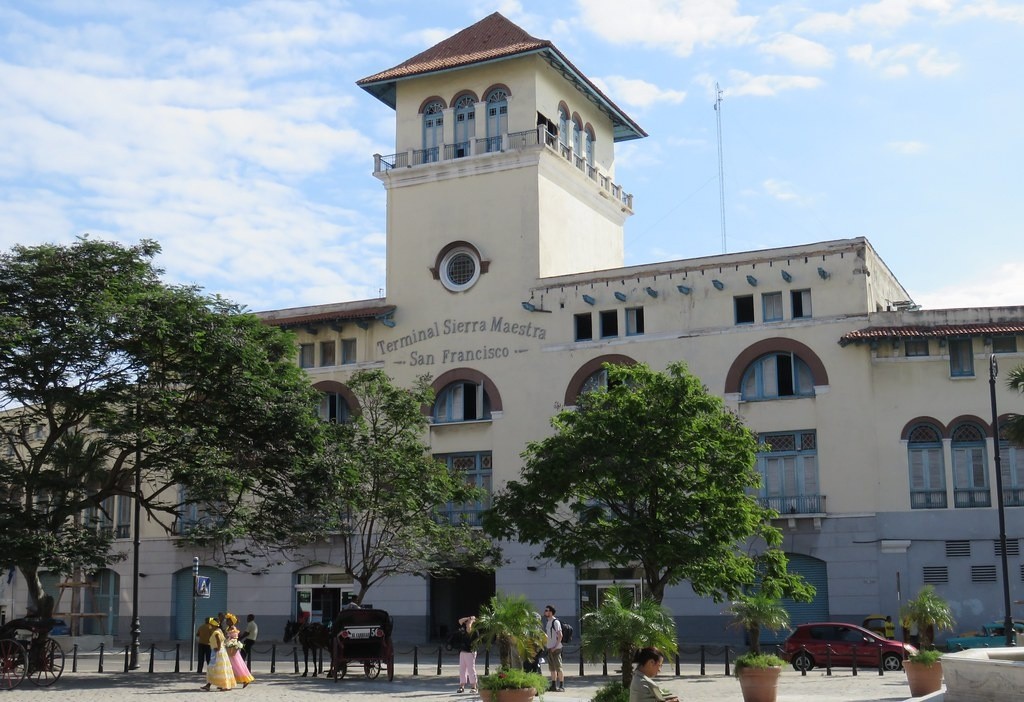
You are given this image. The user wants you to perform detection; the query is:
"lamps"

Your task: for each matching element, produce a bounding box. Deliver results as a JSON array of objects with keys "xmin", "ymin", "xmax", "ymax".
[
  {"xmin": 818, "ymin": 268, "xmax": 830, "ymax": 281},
  {"xmin": 614, "ymin": 292, "xmax": 626, "ymax": 301},
  {"xmin": 781, "ymin": 270, "xmax": 792, "ymax": 283},
  {"xmin": 583, "ymin": 295, "xmax": 595, "ymax": 305},
  {"xmin": 522, "ymin": 296, "xmax": 535, "ymax": 312},
  {"xmin": 303, "ymin": 322, "xmax": 317, "ymax": 335},
  {"xmin": 678, "ymin": 286, "xmax": 690, "ymax": 295},
  {"xmin": 746, "ymin": 275, "xmax": 758, "ymax": 287},
  {"xmin": 711, "ymin": 280, "xmax": 723, "ymax": 290},
  {"xmin": 356, "ymin": 318, "xmax": 369, "ymax": 330},
  {"xmin": 382, "ymin": 316, "xmax": 395, "ymax": 327},
  {"xmin": 327, "ymin": 319, "xmax": 342, "ymax": 332},
  {"xmin": 646, "ymin": 287, "xmax": 657, "ymax": 298}
]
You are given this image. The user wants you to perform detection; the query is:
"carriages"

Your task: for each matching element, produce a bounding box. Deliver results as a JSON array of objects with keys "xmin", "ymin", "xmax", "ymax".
[
  {"xmin": 0, "ymin": 594, "xmax": 64, "ymax": 690},
  {"xmin": 283, "ymin": 605, "xmax": 395, "ymax": 683}
]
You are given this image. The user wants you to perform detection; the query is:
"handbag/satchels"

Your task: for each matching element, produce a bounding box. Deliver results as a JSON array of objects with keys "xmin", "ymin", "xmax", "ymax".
[{"xmin": 449, "ymin": 632, "xmax": 462, "ymax": 650}]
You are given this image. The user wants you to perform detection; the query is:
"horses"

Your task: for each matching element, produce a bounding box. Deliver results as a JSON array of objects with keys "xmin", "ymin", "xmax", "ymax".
[{"xmin": 283, "ymin": 620, "xmax": 334, "ymax": 677}]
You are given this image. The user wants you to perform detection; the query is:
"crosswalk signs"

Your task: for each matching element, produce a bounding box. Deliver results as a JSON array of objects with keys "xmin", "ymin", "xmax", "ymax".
[{"xmin": 197, "ymin": 577, "xmax": 211, "ymax": 599}]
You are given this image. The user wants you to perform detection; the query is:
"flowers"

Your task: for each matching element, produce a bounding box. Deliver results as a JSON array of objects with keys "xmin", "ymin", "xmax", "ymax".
[{"xmin": 476, "ymin": 663, "xmax": 549, "ymax": 702}]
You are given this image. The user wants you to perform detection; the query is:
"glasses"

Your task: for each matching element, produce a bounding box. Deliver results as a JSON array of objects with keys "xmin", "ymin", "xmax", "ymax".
[{"xmin": 545, "ymin": 610, "xmax": 550, "ymax": 612}]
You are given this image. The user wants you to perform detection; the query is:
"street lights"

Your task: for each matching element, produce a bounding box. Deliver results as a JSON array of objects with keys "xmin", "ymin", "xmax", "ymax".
[{"xmin": 989, "ymin": 353, "xmax": 1015, "ymax": 646}]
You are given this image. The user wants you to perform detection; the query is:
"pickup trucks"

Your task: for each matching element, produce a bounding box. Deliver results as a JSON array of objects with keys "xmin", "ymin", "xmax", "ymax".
[{"xmin": 945, "ymin": 622, "xmax": 1024, "ymax": 652}]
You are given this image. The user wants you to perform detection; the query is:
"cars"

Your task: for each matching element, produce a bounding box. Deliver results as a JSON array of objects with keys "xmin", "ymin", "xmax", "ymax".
[{"xmin": 782, "ymin": 622, "xmax": 919, "ymax": 672}]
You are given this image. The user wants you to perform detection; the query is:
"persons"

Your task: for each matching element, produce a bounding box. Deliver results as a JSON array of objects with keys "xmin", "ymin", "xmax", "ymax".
[
  {"xmin": 511, "ymin": 605, "xmax": 565, "ymax": 692},
  {"xmin": 886, "ymin": 611, "xmax": 935, "ymax": 651},
  {"xmin": 457, "ymin": 616, "xmax": 479, "ymax": 694},
  {"xmin": 347, "ymin": 595, "xmax": 362, "ymax": 610},
  {"xmin": 194, "ymin": 612, "xmax": 259, "ymax": 692},
  {"xmin": 629, "ymin": 646, "xmax": 680, "ymax": 702}
]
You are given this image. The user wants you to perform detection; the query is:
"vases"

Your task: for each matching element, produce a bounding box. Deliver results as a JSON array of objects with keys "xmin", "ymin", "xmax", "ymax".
[{"xmin": 478, "ymin": 688, "xmax": 536, "ymax": 702}]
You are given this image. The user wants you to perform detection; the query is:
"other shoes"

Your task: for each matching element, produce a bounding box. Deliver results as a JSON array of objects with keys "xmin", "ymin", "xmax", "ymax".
[
  {"xmin": 558, "ymin": 688, "xmax": 564, "ymax": 692},
  {"xmin": 457, "ymin": 688, "xmax": 464, "ymax": 692},
  {"xmin": 548, "ymin": 687, "xmax": 555, "ymax": 691},
  {"xmin": 470, "ymin": 689, "xmax": 477, "ymax": 693}
]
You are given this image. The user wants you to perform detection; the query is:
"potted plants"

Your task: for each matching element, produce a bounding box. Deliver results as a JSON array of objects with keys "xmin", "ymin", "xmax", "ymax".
[
  {"xmin": 733, "ymin": 651, "xmax": 789, "ymax": 702},
  {"xmin": 902, "ymin": 650, "xmax": 944, "ymax": 697}
]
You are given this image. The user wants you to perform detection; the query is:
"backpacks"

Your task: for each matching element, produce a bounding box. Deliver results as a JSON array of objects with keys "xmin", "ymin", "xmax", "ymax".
[{"xmin": 552, "ymin": 619, "xmax": 573, "ymax": 643}]
